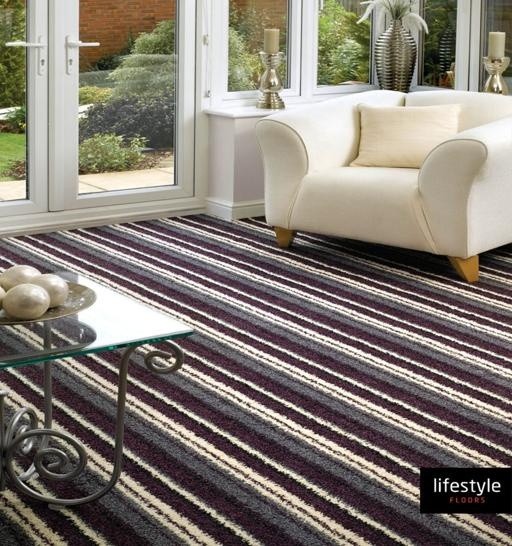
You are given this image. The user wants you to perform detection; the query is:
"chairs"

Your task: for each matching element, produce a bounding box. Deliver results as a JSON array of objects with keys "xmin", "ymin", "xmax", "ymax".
[{"xmin": 255, "ymin": 90, "xmax": 512, "ymax": 284}]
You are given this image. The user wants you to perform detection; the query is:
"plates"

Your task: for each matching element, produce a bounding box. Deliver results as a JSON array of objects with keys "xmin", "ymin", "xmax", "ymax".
[{"xmin": 0, "ymin": 282, "xmax": 97, "ymax": 325}]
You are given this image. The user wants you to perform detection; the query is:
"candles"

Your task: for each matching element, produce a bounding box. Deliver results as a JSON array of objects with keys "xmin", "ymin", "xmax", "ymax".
[
  {"xmin": 264, "ymin": 28, "xmax": 280, "ymax": 54},
  {"xmin": 488, "ymin": 29, "xmax": 505, "ymax": 58}
]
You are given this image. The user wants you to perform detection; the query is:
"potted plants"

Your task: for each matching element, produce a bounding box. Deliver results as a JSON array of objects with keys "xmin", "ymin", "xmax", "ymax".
[
  {"xmin": 423, "ymin": 0, "xmax": 456, "ymax": 85},
  {"xmin": 356, "ymin": 0, "xmax": 429, "ymax": 93}
]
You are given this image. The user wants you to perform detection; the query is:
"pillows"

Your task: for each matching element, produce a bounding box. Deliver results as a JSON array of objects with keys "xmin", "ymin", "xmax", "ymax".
[{"xmin": 350, "ymin": 103, "xmax": 463, "ymax": 167}]
